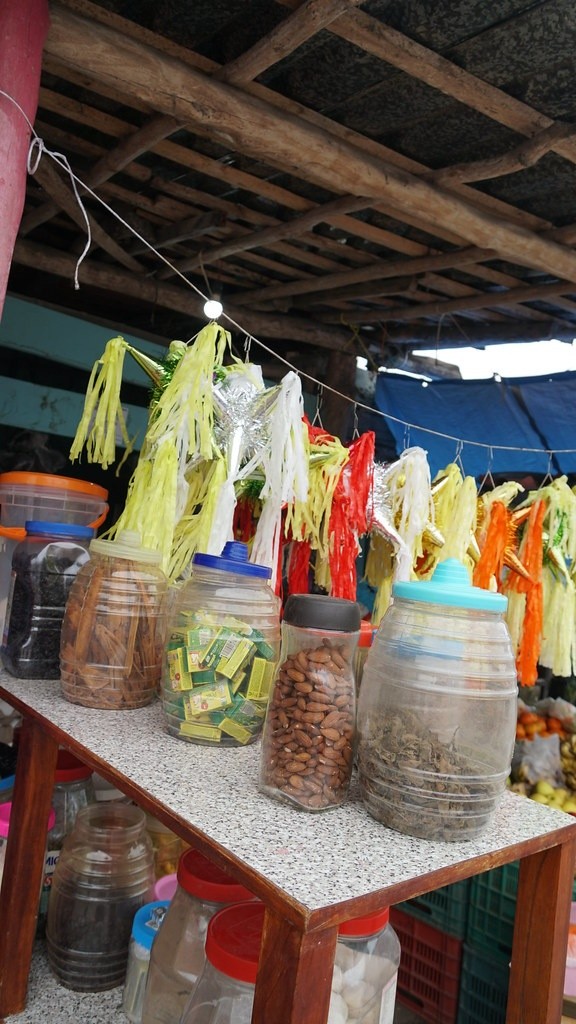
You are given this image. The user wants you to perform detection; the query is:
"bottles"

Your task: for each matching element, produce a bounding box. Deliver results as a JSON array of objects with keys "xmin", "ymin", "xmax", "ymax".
[
  {"xmin": 259, "ymin": 593, "xmax": 364, "ymax": 814},
  {"xmin": 359, "ymin": 557, "xmax": 520, "ymax": 848},
  {"xmin": 54, "ymin": 525, "xmax": 279, "ymax": 746},
  {"xmin": 3, "ymin": 713, "xmax": 403, "ymax": 1023}
]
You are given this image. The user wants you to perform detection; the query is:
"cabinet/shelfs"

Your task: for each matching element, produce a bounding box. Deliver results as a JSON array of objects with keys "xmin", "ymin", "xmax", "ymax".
[{"xmin": 0, "ymin": 656, "xmax": 576, "ymax": 1024}]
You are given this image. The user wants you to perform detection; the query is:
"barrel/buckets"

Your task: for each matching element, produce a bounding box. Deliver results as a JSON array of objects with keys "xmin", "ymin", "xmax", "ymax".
[{"xmin": 0, "ymin": 467, "xmax": 110, "ymax": 711}]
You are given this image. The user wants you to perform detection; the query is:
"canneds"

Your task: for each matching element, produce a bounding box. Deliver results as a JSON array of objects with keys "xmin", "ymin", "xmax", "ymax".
[{"xmin": 260, "ymin": 594, "xmax": 362, "ymax": 813}]
